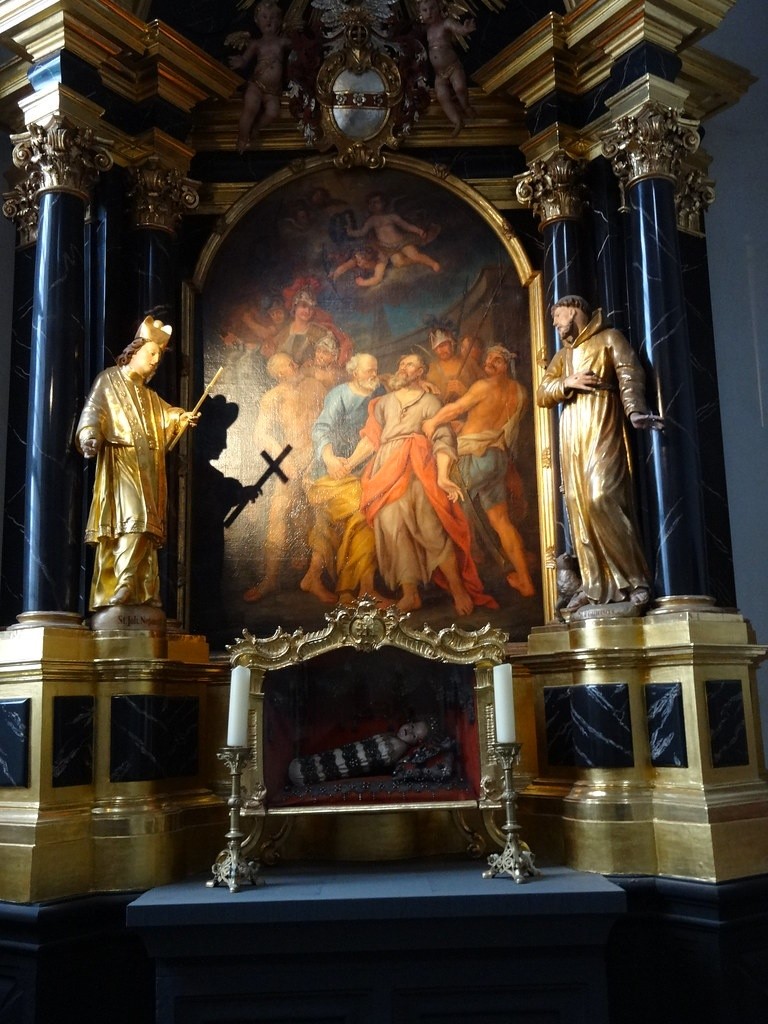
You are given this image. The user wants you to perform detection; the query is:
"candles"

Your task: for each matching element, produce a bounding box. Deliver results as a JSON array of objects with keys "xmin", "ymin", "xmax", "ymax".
[
  {"xmin": 493, "ymin": 662, "xmax": 516, "ymax": 742},
  {"xmin": 224, "ymin": 665, "xmax": 250, "ymax": 747}
]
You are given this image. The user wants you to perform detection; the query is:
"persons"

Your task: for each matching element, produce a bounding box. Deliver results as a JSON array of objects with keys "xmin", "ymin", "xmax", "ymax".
[
  {"xmin": 537, "ymin": 296, "xmax": 666, "ymax": 613},
  {"xmin": 245, "ymin": 783, "xmax": 267, "ymax": 808},
  {"xmin": 76, "ymin": 316, "xmax": 202, "ymax": 612},
  {"xmin": 417, "ymin": 0, "xmax": 478, "ymax": 136},
  {"xmin": 396, "ymin": 714, "xmax": 442, "ymax": 744},
  {"xmin": 228, "ymin": 0, "xmax": 293, "ymax": 155}
]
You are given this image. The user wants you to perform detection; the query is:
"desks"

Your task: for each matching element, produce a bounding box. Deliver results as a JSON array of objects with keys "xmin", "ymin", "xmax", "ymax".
[{"xmin": 126, "ymin": 868, "xmax": 625, "ymax": 1024}]
